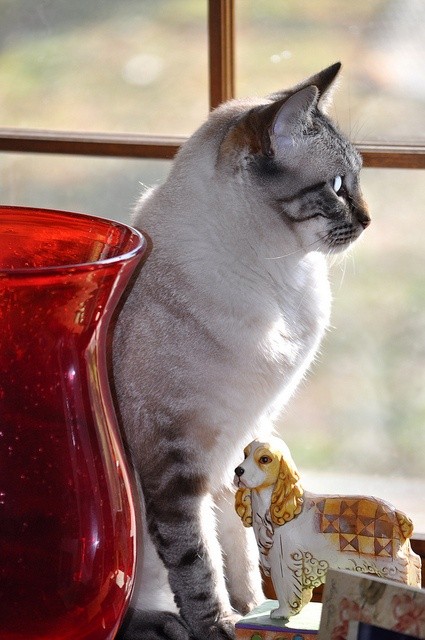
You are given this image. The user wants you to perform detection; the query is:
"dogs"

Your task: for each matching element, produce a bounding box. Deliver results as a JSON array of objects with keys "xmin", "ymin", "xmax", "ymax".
[{"xmin": 233, "ymin": 434, "xmax": 424, "ymax": 621}]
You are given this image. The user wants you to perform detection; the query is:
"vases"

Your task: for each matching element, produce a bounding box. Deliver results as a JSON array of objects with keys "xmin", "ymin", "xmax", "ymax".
[{"xmin": 2, "ymin": 206, "xmax": 152, "ymax": 640}]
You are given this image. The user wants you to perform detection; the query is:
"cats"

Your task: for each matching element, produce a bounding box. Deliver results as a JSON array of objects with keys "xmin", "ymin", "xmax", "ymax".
[{"xmin": 109, "ymin": 58, "xmax": 375, "ymax": 640}]
